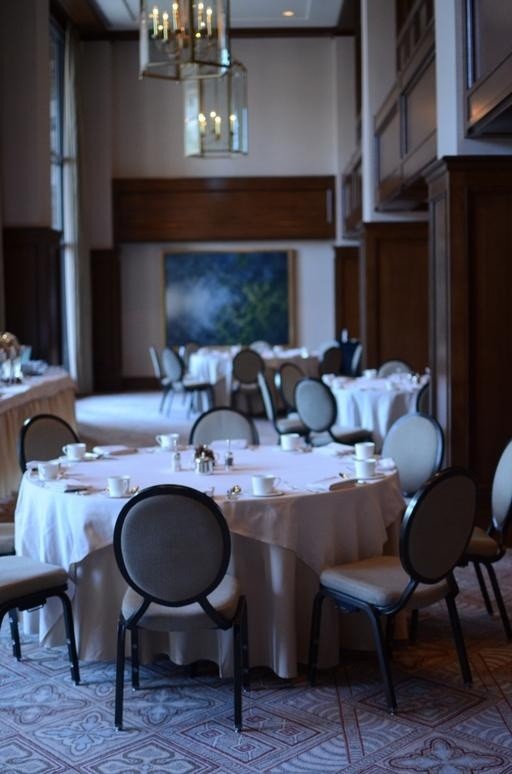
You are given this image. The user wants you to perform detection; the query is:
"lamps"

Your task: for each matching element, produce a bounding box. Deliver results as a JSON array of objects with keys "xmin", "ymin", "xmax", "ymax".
[
  {"xmin": 183, "ymin": 61, "xmax": 250, "ymax": 161},
  {"xmin": 138, "ymin": 1, "xmax": 231, "ymax": 80}
]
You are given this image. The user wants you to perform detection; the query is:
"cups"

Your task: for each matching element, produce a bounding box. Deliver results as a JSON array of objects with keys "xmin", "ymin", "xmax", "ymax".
[
  {"xmin": 355, "ymin": 459, "xmax": 376, "ymax": 480},
  {"xmin": 108, "ymin": 475, "xmax": 130, "ymax": 498},
  {"xmin": 355, "ymin": 443, "xmax": 374, "ymax": 459},
  {"xmin": 252, "ymin": 475, "xmax": 281, "ymax": 496},
  {"xmin": 281, "ymin": 434, "xmax": 299, "ymax": 450},
  {"xmin": 156, "ymin": 434, "xmax": 179, "ymax": 452},
  {"xmin": 63, "ymin": 443, "xmax": 86, "ymax": 461},
  {"xmin": 38, "ymin": 462, "xmax": 58, "ymax": 481}
]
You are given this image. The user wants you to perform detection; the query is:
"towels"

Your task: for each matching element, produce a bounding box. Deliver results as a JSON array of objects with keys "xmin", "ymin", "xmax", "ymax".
[
  {"xmin": 45, "ymin": 477, "xmax": 90, "ymax": 495},
  {"xmin": 313, "ymin": 441, "xmax": 354, "ymax": 458}
]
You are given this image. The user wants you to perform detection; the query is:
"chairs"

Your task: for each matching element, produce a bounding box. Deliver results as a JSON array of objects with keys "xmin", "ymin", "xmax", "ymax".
[
  {"xmin": 275, "ymin": 363, "xmax": 306, "ymax": 409},
  {"xmin": 188, "ymin": 407, "xmax": 260, "ymax": 447},
  {"xmin": 305, "ymin": 467, "xmax": 472, "ymax": 715},
  {"xmin": 228, "ymin": 350, "xmax": 271, "ymax": 419},
  {"xmin": 408, "ymin": 441, "xmax": 512, "ymax": 642},
  {"xmin": 382, "ymin": 412, "xmax": 445, "ymax": 496},
  {"xmin": 377, "ymin": 360, "xmax": 408, "ymax": 375},
  {"xmin": 149, "ymin": 346, "xmax": 170, "ymax": 415},
  {"xmin": 0, "ymin": 555, "xmax": 81, "ymax": 686},
  {"xmin": 15, "ymin": 413, "xmax": 81, "ymax": 473},
  {"xmin": 351, "ymin": 341, "xmax": 363, "ymax": 375},
  {"xmin": 255, "ymin": 368, "xmax": 311, "ymax": 446},
  {"xmin": 319, "ymin": 348, "xmax": 344, "ymax": 377},
  {"xmin": 178, "ymin": 340, "xmax": 201, "ymax": 365},
  {"xmin": 293, "ymin": 378, "xmax": 371, "ymax": 445},
  {"xmin": 248, "ymin": 341, "xmax": 275, "ymax": 353},
  {"xmin": 111, "ymin": 483, "xmax": 249, "ymax": 734},
  {"xmin": 161, "ymin": 347, "xmax": 216, "ymax": 419}
]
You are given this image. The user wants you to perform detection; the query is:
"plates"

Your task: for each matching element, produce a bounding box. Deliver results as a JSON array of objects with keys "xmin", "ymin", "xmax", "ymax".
[{"xmin": 370, "ymin": 472, "xmax": 384, "ymax": 479}]
